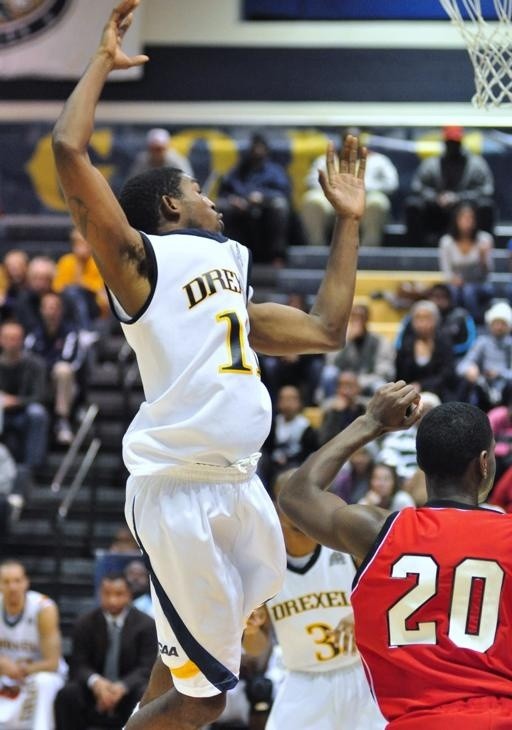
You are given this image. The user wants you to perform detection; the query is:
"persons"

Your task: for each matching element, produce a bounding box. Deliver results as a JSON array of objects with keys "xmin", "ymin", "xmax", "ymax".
[
  {"xmin": 0, "ymin": 250, "xmax": 27, "ymax": 323},
  {"xmin": 279, "ymin": 380, "xmax": 512, "ymax": 730},
  {"xmin": 299, "ymin": 125, "xmax": 400, "ymax": 247},
  {"xmin": 0, "ymin": 323, "xmax": 51, "ymax": 509},
  {"xmin": 455, "ymin": 299, "xmax": 512, "ymax": 413},
  {"xmin": 0, "ymin": 564, "xmax": 68, "ymax": 730},
  {"xmin": 124, "ymin": 128, "xmax": 193, "ymax": 178},
  {"xmin": 379, "ymin": 392, "xmax": 443, "ymax": 503},
  {"xmin": 54, "ymin": 571, "xmax": 159, "ymax": 730},
  {"xmin": 111, "ymin": 528, "xmax": 139, "ymax": 555},
  {"xmin": 394, "ymin": 298, "xmax": 457, "ymax": 404},
  {"xmin": 356, "ymin": 460, "xmax": 417, "ymax": 511},
  {"xmin": 320, "ymin": 371, "xmax": 370, "ymax": 446},
  {"xmin": 122, "ymin": 559, "xmax": 154, "ymax": 616},
  {"xmin": 242, "ymin": 463, "xmax": 390, "ymax": 730},
  {"xmin": 394, "ymin": 284, "xmax": 477, "ymax": 359},
  {"xmin": 216, "ymin": 131, "xmax": 294, "ymax": 267},
  {"xmin": 24, "ymin": 292, "xmax": 89, "ymax": 445},
  {"xmin": 238, "ymin": 605, "xmax": 277, "ymax": 730},
  {"xmin": 321, "ymin": 305, "xmax": 393, "ymax": 396},
  {"xmin": 438, "ymin": 198, "xmax": 495, "ymax": 324},
  {"xmin": 16, "ymin": 256, "xmax": 54, "ymax": 333},
  {"xmin": 487, "ymin": 405, "xmax": 512, "ymax": 460},
  {"xmin": 1, "ymin": 440, "xmax": 23, "ymax": 556},
  {"xmin": 51, "ymin": 0, "xmax": 368, "ymax": 730},
  {"xmin": 405, "ymin": 125, "xmax": 496, "ymax": 247},
  {"xmin": 273, "ymin": 287, "xmax": 317, "ymax": 405},
  {"xmin": 52, "ymin": 226, "xmax": 111, "ymax": 360},
  {"xmin": 257, "ymin": 382, "xmax": 320, "ymax": 500}
]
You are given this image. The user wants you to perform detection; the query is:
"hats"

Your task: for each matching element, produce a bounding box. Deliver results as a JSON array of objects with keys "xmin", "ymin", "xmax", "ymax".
[
  {"xmin": 442, "ymin": 126, "xmax": 463, "ymax": 143},
  {"xmin": 485, "ymin": 302, "xmax": 511, "ymax": 325}
]
